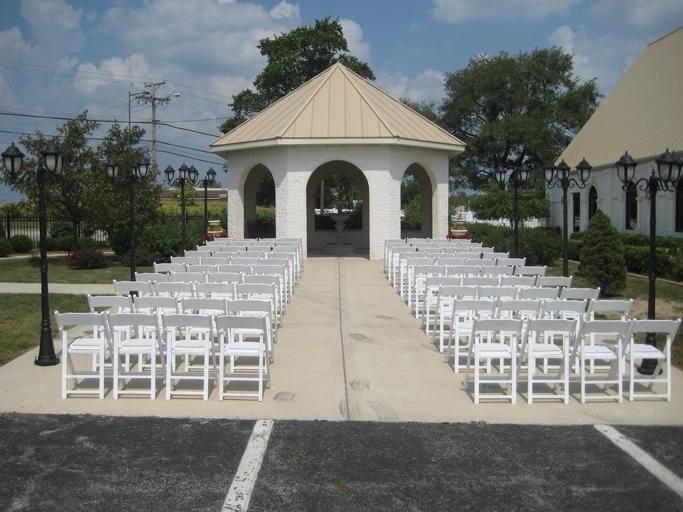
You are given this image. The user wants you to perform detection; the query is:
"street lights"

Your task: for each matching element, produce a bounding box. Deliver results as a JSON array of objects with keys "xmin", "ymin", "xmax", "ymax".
[
  {"xmin": 495, "ymin": 164, "xmax": 530, "ymax": 258},
  {"xmin": 615, "ymin": 148, "xmax": 683, "ymax": 374},
  {"xmin": 104, "ymin": 156, "xmax": 152, "ymax": 301},
  {"xmin": 163, "ymin": 161, "xmax": 216, "ymax": 257},
  {"xmin": 543, "ymin": 157, "xmax": 592, "ymax": 277},
  {"xmin": 3, "ymin": 141, "xmax": 66, "ymax": 366}
]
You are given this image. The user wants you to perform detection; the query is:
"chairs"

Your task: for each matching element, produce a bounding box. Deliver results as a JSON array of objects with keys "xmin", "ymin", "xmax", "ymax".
[
  {"xmin": 382, "ymin": 235, "xmax": 682, "ymax": 407},
  {"xmin": 58, "ymin": 236, "xmax": 302, "ymax": 402}
]
